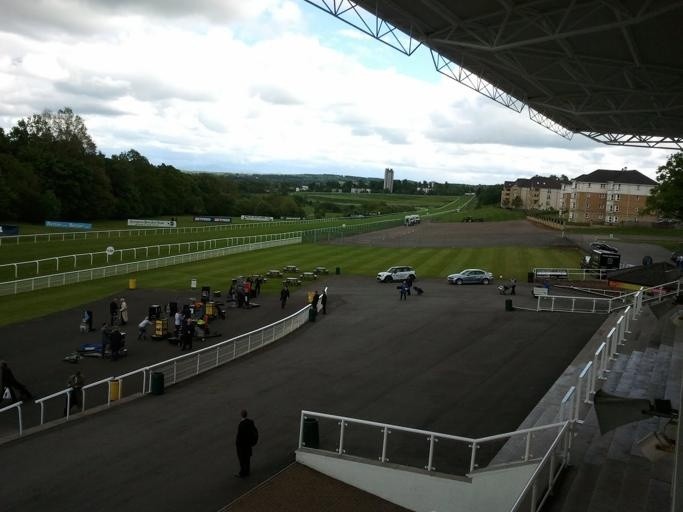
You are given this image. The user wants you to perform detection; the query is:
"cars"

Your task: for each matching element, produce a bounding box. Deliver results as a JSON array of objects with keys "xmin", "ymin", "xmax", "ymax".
[
  {"xmin": 580, "ymin": 255, "xmax": 591, "ymax": 271},
  {"xmin": 447, "ymin": 268, "xmax": 493, "ymax": 285},
  {"xmin": 657, "ymin": 214, "xmax": 681, "ymax": 225},
  {"xmin": 589, "ymin": 243, "xmax": 618, "ymax": 253}
]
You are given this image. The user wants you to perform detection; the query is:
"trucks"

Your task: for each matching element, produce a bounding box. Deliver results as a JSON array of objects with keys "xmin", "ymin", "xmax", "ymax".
[
  {"xmin": 404, "ymin": 214, "xmax": 420, "ymax": 226},
  {"xmin": 589, "ymin": 249, "xmax": 621, "ymax": 279}
]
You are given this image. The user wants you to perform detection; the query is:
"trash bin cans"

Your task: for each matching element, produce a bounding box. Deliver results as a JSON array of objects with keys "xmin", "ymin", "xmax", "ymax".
[
  {"xmin": 303, "ymin": 416, "xmax": 320, "ymax": 451},
  {"xmin": 128, "ymin": 278, "xmax": 137, "ymax": 289},
  {"xmin": 336, "ymin": 267, "xmax": 340, "ymax": 275},
  {"xmin": 505, "ymin": 299, "xmax": 512, "ymax": 312},
  {"xmin": 527, "ymin": 272, "xmax": 534, "ymax": 283},
  {"xmin": 309, "ymin": 310, "xmax": 317, "ymax": 323},
  {"xmin": 152, "ymin": 372, "xmax": 165, "ymax": 397},
  {"xmin": 109, "ymin": 379, "xmax": 120, "ymax": 402}
]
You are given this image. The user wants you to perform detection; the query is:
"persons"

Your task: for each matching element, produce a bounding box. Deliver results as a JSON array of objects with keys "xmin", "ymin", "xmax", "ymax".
[
  {"xmin": 100, "ymin": 322, "xmax": 112, "ymax": 355},
  {"xmin": 255, "ymin": 276, "xmax": 262, "ymax": 296},
  {"xmin": 232, "ymin": 409, "xmax": 257, "ymax": 478},
  {"xmin": 136, "ymin": 315, "xmax": 152, "ymax": 341},
  {"xmin": 510, "ymin": 280, "xmax": 517, "ymax": 295},
  {"xmin": 63, "ymin": 368, "xmax": 85, "ymax": 418},
  {"xmin": 321, "ymin": 291, "xmax": 327, "ymax": 314},
  {"xmin": 109, "ymin": 297, "xmax": 121, "ymax": 327},
  {"xmin": 279, "ymin": 287, "xmax": 290, "ymax": 309},
  {"xmin": 413, "ymin": 286, "xmax": 424, "ymax": 296},
  {"xmin": 179, "ymin": 317, "xmax": 195, "ymax": 351},
  {"xmin": 312, "ymin": 290, "xmax": 320, "ymax": 316},
  {"xmin": 407, "ymin": 276, "xmax": 412, "ymax": 297},
  {"xmin": 173, "ymin": 308, "xmax": 185, "ymax": 338},
  {"xmin": 118, "ymin": 298, "xmax": 129, "ymax": 327},
  {"xmin": 543, "ymin": 278, "xmax": 550, "ymax": 293},
  {"xmin": 400, "ymin": 279, "xmax": 409, "ymax": 301},
  {"xmin": 108, "ymin": 326, "xmax": 122, "ymax": 362}
]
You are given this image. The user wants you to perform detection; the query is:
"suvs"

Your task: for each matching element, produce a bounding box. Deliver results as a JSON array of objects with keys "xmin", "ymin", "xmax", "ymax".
[{"xmin": 375, "ymin": 266, "xmax": 416, "ymax": 282}]
